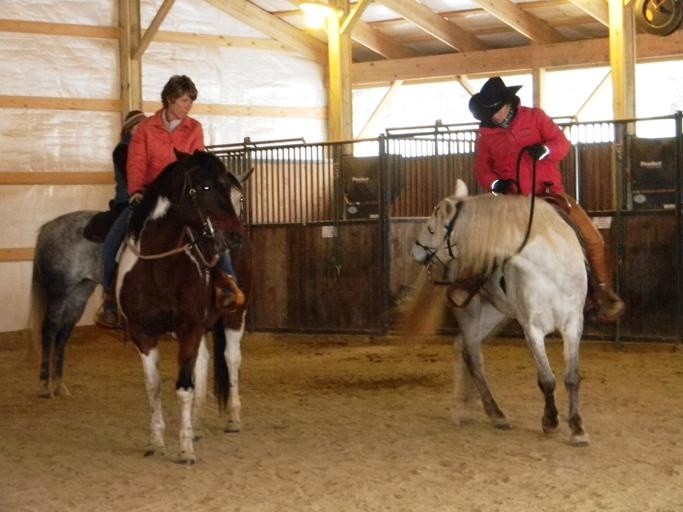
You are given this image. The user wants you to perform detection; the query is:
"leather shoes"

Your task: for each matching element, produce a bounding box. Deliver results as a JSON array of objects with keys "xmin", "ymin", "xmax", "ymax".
[
  {"xmin": 104, "ymin": 304, "xmax": 117, "ymax": 324},
  {"xmin": 598, "ymin": 286, "xmax": 624, "ymax": 315},
  {"xmin": 221, "ymin": 287, "xmax": 235, "ymax": 307}
]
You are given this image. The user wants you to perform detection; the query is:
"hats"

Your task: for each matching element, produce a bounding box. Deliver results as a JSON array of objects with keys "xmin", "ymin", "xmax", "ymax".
[
  {"xmin": 469, "ymin": 76, "xmax": 523, "ymax": 120},
  {"xmin": 123, "ymin": 110, "xmax": 146, "ymax": 128}
]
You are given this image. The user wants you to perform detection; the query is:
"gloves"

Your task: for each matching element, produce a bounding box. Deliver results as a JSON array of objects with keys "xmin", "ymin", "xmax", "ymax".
[
  {"xmin": 492, "ymin": 180, "xmax": 513, "ymax": 192},
  {"xmin": 528, "ymin": 144, "xmax": 550, "ymax": 160}
]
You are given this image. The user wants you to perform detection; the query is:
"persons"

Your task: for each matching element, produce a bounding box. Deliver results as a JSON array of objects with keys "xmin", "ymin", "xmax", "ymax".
[
  {"xmin": 469, "ymin": 73, "xmax": 625, "ymax": 324},
  {"xmin": 111, "ymin": 110, "xmax": 147, "ymax": 203},
  {"xmin": 92, "ymin": 75, "xmax": 244, "ymax": 326}
]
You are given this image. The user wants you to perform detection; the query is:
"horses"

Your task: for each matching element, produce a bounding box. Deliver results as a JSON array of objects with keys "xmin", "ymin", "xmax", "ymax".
[
  {"xmin": 406, "ymin": 177, "xmax": 593, "ymax": 448},
  {"xmin": 115, "ymin": 148, "xmax": 245, "ymax": 464},
  {"xmin": 27, "ymin": 167, "xmax": 253, "ymax": 397}
]
